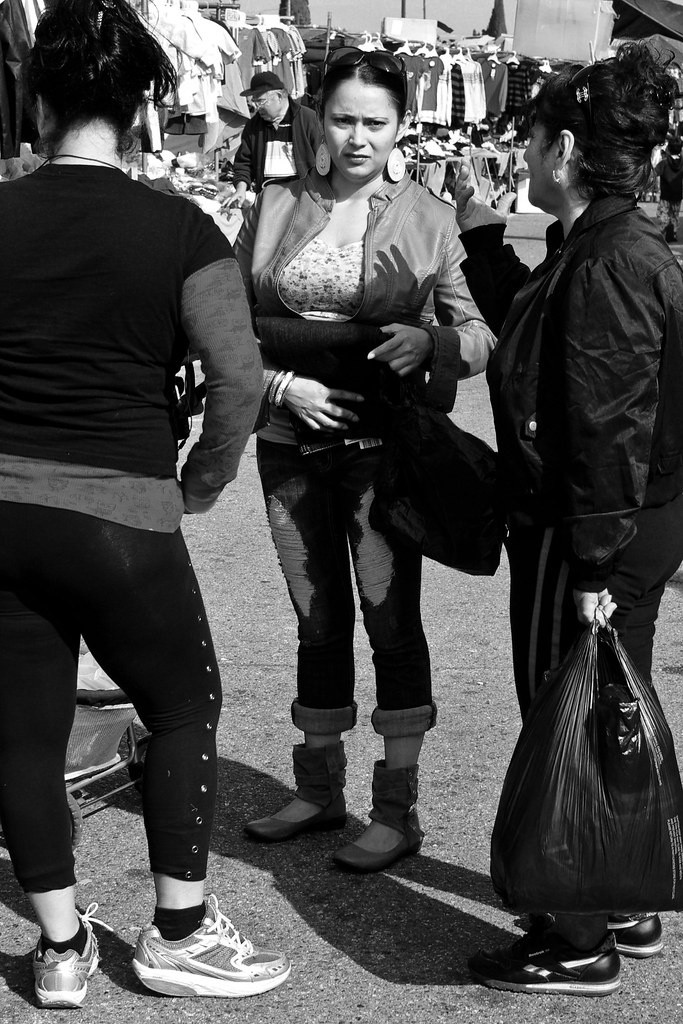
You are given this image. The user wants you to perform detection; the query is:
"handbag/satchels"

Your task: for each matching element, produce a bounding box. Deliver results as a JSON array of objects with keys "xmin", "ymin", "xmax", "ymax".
[{"xmin": 370, "ymin": 322, "xmax": 504, "ymax": 575}]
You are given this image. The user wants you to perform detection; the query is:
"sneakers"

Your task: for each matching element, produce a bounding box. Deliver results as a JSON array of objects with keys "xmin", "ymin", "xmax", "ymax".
[
  {"xmin": 31, "ymin": 902, "xmax": 114, "ymax": 1007},
  {"xmin": 468, "ymin": 934, "xmax": 621, "ymax": 996},
  {"xmin": 608, "ymin": 914, "xmax": 665, "ymax": 956},
  {"xmin": 133, "ymin": 894, "xmax": 291, "ymax": 996}
]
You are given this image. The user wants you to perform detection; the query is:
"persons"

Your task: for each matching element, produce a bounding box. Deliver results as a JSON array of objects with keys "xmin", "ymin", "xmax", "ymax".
[
  {"xmin": 639, "ymin": 139, "xmax": 668, "ymax": 202},
  {"xmin": 221, "ymin": 70, "xmax": 323, "ymax": 208},
  {"xmin": 653, "ymin": 137, "xmax": 683, "ymax": 242},
  {"xmin": 0, "ymin": 0, "xmax": 291, "ymax": 1009},
  {"xmin": 455, "ymin": 46, "xmax": 683, "ymax": 995},
  {"xmin": 232, "ymin": 51, "xmax": 498, "ymax": 872}
]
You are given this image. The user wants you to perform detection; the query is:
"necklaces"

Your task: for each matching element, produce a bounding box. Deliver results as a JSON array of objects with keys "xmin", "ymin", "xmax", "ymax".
[{"xmin": 35, "ymin": 154, "xmax": 120, "ymax": 170}]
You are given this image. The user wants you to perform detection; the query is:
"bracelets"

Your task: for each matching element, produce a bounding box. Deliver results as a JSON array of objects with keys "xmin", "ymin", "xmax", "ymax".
[{"xmin": 269, "ymin": 371, "xmax": 295, "ymax": 406}]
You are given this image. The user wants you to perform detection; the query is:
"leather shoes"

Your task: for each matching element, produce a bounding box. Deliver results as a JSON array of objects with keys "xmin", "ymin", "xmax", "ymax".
[
  {"xmin": 333, "ymin": 806, "xmax": 425, "ymax": 870},
  {"xmin": 242, "ymin": 798, "xmax": 345, "ymax": 843}
]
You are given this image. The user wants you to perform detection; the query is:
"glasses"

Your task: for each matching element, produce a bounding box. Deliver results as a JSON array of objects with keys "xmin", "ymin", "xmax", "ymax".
[
  {"xmin": 568, "ymin": 66, "xmax": 598, "ymax": 141},
  {"xmin": 245, "ymin": 96, "xmax": 271, "ymax": 110},
  {"xmin": 325, "ymin": 47, "xmax": 406, "ymax": 77}
]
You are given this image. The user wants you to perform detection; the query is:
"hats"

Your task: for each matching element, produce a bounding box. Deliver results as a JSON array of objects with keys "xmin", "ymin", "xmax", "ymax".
[{"xmin": 240, "ymin": 70, "xmax": 285, "ymax": 97}]
